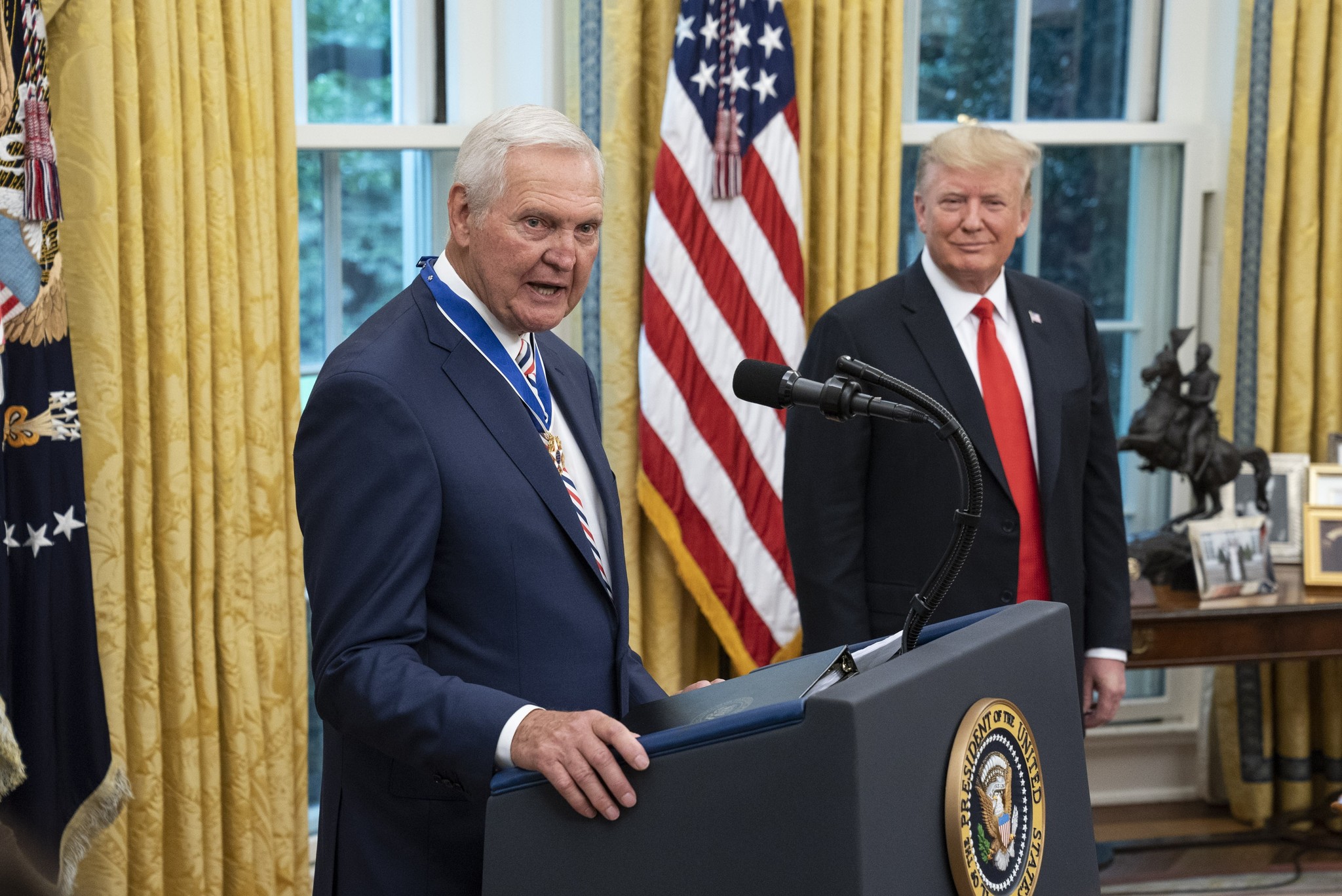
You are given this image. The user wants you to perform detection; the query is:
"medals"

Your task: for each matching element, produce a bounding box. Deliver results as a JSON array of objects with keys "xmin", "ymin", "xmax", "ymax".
[{"xmin": 547, "ymin": 435, "xmax": 566, "ymax": 476}]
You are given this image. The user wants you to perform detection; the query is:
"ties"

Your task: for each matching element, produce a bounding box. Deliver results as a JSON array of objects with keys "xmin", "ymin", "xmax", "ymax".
[
  {"xmin": 518, "ymin": 342, "xmax": 610, "ymax": 586},
  {"xmin": 971, "ymin": 297, "xmax": 1052, "ymax": 602}
]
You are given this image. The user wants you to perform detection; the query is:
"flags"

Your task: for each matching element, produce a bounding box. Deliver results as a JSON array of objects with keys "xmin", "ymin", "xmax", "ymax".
[
  {"xmin": 0, "ymin": 2, "xmax": 134, "ymax": 896},
  {"xmin": 638, "ymin": 2, "xmax": 804, "ymax": 677}
]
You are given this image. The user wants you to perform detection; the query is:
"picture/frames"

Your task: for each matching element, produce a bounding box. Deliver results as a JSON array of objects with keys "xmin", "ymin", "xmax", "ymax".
[
  {"xmin": 1303, "ymin": 505, "xmax": 1342, "ymax": 587},
  {"xmin": 1327, "ymin": 432, "xmax": 1342, "ymax": 463},
  {"xmin": 1218, "ymin": 453, "xmax": 1309, "ymax": 563},
  {"xmin": 1188, "ymin": 517, "xmax": 1275, "ymax": 597},
  {"xmin": 1309, "ymin": 463, "xmax": 1342, "ymax": 506}
]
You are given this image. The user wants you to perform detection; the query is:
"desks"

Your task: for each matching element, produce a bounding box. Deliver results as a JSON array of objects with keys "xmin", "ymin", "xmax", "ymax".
[{"xmin": 1109, "ymin": 571, "xmax": 1342, "ymax": 856}]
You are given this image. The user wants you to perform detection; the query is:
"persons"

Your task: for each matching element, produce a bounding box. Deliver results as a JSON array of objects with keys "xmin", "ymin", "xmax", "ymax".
[
  {"xmin": 785, "ymin": 126, "xmax": 1130, "ymax": 742},
  {"xmin": 1137, "ymin": 342, "xmax": 1221, "ymax": 474},
  {"xmin": 293, "ymin": 103, "xmax": 727, "ymax": 896},
  {"xmin": 1217, "ymin": 533, "xmax": 1255, "ymax": 583}
]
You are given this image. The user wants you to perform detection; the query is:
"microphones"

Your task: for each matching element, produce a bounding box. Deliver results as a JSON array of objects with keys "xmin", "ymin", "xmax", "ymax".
[{"xmin": 732, "ymin": 358, "xmax": 929, "ymax": 424}]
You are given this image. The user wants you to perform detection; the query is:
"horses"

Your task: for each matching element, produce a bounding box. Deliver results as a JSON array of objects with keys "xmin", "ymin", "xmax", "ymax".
[{"xmin": 1117, "ymin": 325, "xmax": 1271, "ymax": 534}]
